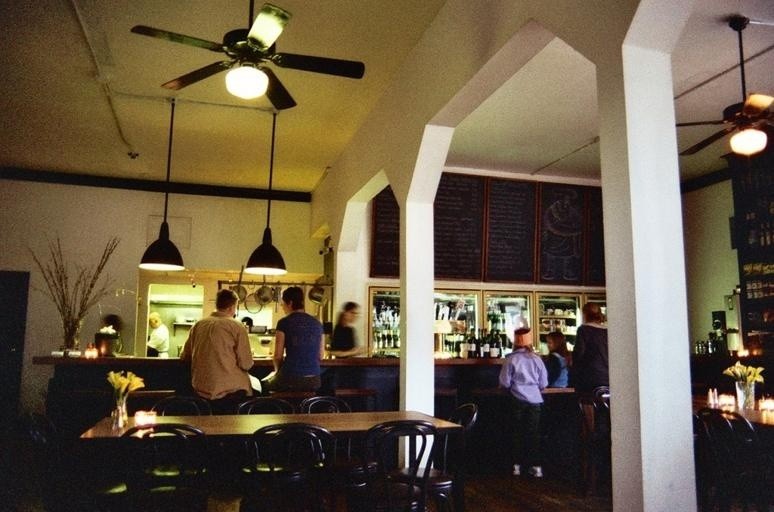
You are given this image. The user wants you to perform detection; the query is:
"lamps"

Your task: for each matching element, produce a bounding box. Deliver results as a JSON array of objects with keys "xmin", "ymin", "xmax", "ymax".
[
  {"xmin": 222, "ymin": 55, "xmax": 270, "ymax": 103},
  {"xmin": 723, "ymin": 14, "xmax": 767, "ymax": 158},
  {"xmin": 137, "ymin": 92, "xmax": 189, "ymax": 272},
  {"xmin": 239, "ymin": 105, "xmax": 287, "ymax": 275}
]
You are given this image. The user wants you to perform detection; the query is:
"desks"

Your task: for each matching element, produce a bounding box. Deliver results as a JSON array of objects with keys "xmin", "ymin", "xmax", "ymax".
[{"xmin": 80, "ymin": 408, "xmax": 466, "ymax": 439}]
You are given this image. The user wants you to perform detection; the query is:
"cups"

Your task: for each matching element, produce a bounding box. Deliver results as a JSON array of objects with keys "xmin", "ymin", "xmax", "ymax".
[
  {"xmin": 135, "ymin": 411, "xmax": 157, "ymax": 427},
  {"xmin": 717, "ymin": 394, "xmax": 774, "ymax": 412},
  {"xmin": 94, "ymin": 332, "xmax": 122, "ymax": 356}
]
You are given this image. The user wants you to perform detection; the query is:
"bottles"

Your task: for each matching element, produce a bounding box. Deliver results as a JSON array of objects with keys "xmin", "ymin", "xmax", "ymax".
[
  {"xmin": 742, "ymin": 207, "xmax": 773, "ymax": 247},
  {"xmin": 694, "ymin": 339, "xmax": 716, "ymax": 354},
  {"xmin": 707, "ymin": 388, "xmax": 718, "ymax": 406},
  {"xmin": 371, "ymin": 304, "xmax": 503, "ymax": 362}
]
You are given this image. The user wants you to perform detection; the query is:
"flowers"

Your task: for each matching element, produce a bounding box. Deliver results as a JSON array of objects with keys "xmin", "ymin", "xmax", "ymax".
[{"xmin": 105, "ymin": 368, "xmax": 146, "ymax": 391}]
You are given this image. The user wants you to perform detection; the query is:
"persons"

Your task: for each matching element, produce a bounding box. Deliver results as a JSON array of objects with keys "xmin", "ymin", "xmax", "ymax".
[
  {"xmin": 541, "ymin": 332, "xmax": 574, "ymax": 389},
  {"xmin": 326, "ymin": 301, "xmax": 361, "ymax": 358},
  {"xmin": 179, "ymin": 289, "xmax": 259, "ymax": 471},
  {"xmin": 147, "ymin": 312, "xmax": 169, "ymax": 358},
  {"xmin": 571, "ymin": 301, "xmax": 608, "ymax": 429},
  {"xmin": 98, "ymin": 314, "xmax": 125, "ymax": 355},
  {"xmin": 261, "ymin": 287, "xmax": 322, "ymax": 463},
  {"xmin": 499, "ymin": 327, "xmax": 549, "ymax": 479}
]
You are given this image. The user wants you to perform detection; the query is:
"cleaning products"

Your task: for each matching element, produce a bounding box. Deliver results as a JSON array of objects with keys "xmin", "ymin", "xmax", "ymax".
[{"xmin": 728, "ymin": 296, "xmax": 733, "ymax": 310}]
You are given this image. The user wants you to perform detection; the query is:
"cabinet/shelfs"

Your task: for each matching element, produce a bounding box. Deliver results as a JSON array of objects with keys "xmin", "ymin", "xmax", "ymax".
[{"xmin": 368, "ymin": 285, "xmax": 609, "ymax": 356}]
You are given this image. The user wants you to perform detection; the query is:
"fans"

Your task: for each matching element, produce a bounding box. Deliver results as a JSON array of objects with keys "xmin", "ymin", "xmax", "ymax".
[
  {"xmin": 126, "ymin": 1, "xmax": 368, "ymax": 111},
  {"xmin": 669, "ymin": 87, "xmax": 773, "ymax": 170}
]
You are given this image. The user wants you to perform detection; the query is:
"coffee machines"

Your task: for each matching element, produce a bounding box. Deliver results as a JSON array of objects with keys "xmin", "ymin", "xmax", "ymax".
[{"xmin": 709, "ymin": 309, "xmax": 727, "ymax": 353}]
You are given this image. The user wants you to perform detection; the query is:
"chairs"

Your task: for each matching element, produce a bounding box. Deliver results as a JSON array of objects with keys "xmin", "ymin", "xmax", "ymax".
[
  {"xmin": 113, "ymin": 423, "xmax": 214, "ymax": 512},
  {"xmin": 152, "ymin": 395, "xmax": 215, "ymax": 417},
  {"xmin": 236, "ymin": 396, "xmax": 297, "ymax": 413},
  {"xmin": 538, "ymin": 378, "xmax": 772, "ymax": 511},
  {"xmin": 299, "ymin": 396, "xmax": 352, "ymax": 413},
  {"xmin": 345, "ymin": 418, "xmax": 437, "ymax": 512},
  {"xmin": 236, "ymin": 422, "xmax": 338, "ymax": 512},
  {"xmin": 423, "ymin": 403, "xmax": 479, "ymax": 511}
]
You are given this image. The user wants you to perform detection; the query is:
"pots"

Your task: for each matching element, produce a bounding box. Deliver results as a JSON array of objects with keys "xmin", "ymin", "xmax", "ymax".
[{"xmin": 227, "ymin": 265, "xmax": 325, "ymax": 319}]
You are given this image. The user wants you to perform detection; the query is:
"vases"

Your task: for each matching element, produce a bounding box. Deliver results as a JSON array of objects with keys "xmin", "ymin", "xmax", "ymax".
[{"xmin": 115, "ymin": 399, "xmax": 129, "ymax": 429}]
